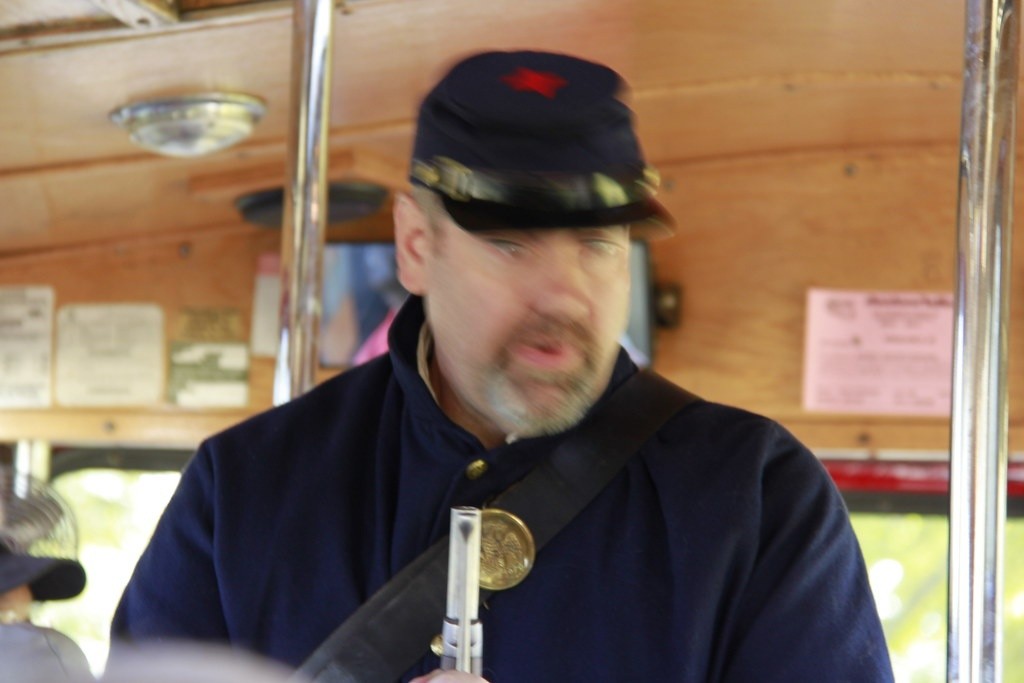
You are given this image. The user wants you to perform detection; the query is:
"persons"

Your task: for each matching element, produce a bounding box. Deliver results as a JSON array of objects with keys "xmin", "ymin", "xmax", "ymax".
[
  {"xmin": 103, "ymin": 50, "xmax": 896, "ymax": 683},
  {"xmin": 0, "ymin": 540, "xmax": 91, "ymax": 683}
]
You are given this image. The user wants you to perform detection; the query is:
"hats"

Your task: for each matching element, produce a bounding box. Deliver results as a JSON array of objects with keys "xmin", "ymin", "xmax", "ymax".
[
  {"xmin": 410, "ymin": 51, "xmax": 659, "ymax": 233},
  {"xmin": 0, "ymin": 537, "xmax": 86, "ymax": 601}
]
